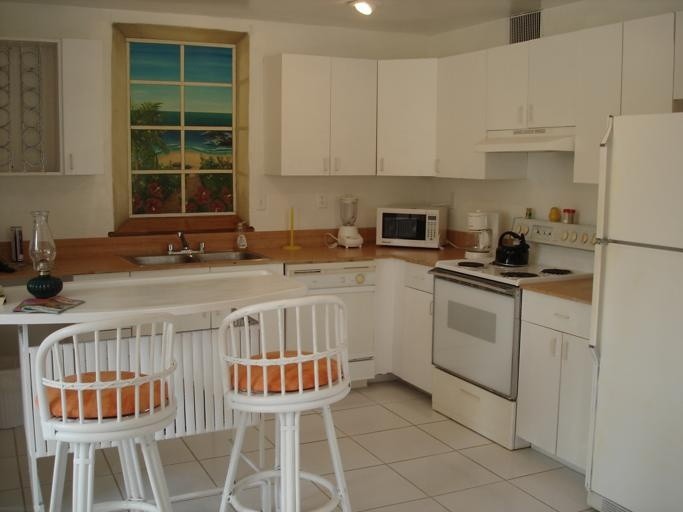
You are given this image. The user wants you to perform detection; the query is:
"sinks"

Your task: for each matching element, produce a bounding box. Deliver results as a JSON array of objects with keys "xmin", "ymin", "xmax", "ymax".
[
  {"xmin": 134, "ymin": 254, "xmax": 197, "ymax": 265},
  {"xmin": 196, "ymin": 249, "xmax": 264, "ymax": 261}
]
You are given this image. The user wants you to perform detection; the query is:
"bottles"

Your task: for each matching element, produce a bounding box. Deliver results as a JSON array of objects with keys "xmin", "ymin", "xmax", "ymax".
[
  {"xmin": 8, "ymin": 226, "xmax": 24, "ymax": 264},
  {"xmin": 562, "ymin": 209, "xmax": 575, "ymax": 225},
  {"xmin": 525, "ymin": 208, "xmax": 532, "ymax": 219},
  {"xmin": 549, "ymin": 207, "xmax": 560, "ymax": 222},
  {"xmin": 28, "ymin": 211, "xmax": 55, "ymax": 271},
  {"xmin": 234, "ymin": 222, "xmax": 247, "ymax": 253}
]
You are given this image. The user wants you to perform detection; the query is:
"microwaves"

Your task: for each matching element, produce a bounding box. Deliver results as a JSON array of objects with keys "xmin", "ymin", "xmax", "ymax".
[{"xmin": 376, "ymin": 205, "xmax": 447, "ymax": 248}]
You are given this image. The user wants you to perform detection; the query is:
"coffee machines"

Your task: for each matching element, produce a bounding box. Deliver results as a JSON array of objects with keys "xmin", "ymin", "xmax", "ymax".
[{"xmin": 461, "ymin": 209, "xmax": 500, "ymax": 264}]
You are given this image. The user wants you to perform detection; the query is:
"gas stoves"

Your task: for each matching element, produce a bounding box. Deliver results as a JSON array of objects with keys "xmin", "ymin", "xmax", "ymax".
[{"xmin": 436, "ymin": 256, "xmax": 585, "ymax": 288}]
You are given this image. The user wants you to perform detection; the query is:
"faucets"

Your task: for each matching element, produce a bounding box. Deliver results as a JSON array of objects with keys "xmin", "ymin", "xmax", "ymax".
[{"xmin": 176, "ymin": 231, "xmax": 188, "ymax": 247}]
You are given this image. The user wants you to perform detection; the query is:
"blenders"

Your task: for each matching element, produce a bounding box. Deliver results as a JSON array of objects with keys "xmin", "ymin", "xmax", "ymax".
[{"xmin": 335, "ymin": 193, "xmax": 364, "ymax": 247}]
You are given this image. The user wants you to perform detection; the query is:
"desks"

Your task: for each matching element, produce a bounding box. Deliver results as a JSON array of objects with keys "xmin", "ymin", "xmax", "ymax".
[{"xmin": 0, "ymin": 273, "xmax": 311, "ymax": 510}]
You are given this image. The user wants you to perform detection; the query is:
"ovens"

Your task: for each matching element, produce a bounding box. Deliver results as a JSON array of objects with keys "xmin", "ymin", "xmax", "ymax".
[{"xmin": 429, "ymin": 267, "xmax": 530, "ymax": 451}]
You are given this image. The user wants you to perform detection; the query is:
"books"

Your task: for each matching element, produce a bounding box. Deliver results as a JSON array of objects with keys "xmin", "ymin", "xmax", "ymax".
[{"xmin": 21, "ymin": 296, "xmax": 85, "ymax": 314}]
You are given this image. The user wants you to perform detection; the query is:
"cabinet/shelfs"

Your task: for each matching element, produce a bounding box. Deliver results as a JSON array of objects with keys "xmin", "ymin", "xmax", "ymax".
[
  {"xmin": 72, "ymin": 263, "xmax": 287, "ymax": 284},
  {"xmin": 261, "ymin": 52, "xmax": 375, "ymax": 178},
  {"xmin": 432, "ymin": 48, "xmax": 527, "ymax": 182},
  {"xmin": 482, "ymin": 28, "xmax": 582, "ymax": 133},
  {"xmin": 0, "ymin": 34, "xmax": 108, "ymax": 178},
  {"xmin": 570, "ymin": 10, "xmax": 682, "ymax": 186},
  {"xmin": 429, "ymin": 367, "xmax": 531, "ymax": 452},
  {"xmin": 513, "ymin": 289, "xmax": 596, "ymax": 474},
  {"xmin": 373, "ymin": 58, "xmax": 436, "ymax": 178},
  {"xmin": 392, "ymin": 253, "xmax": 433, "ymax": 398}
]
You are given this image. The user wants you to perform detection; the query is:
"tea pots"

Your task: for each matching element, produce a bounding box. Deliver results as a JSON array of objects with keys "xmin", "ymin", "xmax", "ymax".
[{"xmin": 494, "ymin": 230, "xmax": 530, "ymax": 267}]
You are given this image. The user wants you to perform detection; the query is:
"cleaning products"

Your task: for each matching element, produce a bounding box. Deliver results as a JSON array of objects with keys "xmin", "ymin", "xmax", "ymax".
[{"xmin": 233, "ymin": 221, "xmax": 249, "ymax": 252}]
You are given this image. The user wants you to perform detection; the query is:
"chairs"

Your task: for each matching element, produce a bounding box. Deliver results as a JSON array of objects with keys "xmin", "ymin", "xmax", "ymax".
[
  {"xmin": 33, "ymin": 310, "xmax": 176, "ymax": 512},
  {"xmin": 211, "ymin": 292, "xmax": 354, "ymax": 511}
]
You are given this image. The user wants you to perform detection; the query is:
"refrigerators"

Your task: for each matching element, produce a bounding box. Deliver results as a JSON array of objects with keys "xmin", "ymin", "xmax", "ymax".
[{"xmin": 579, "ymin": 109, "xmax": 682, "ymax": 511}]
